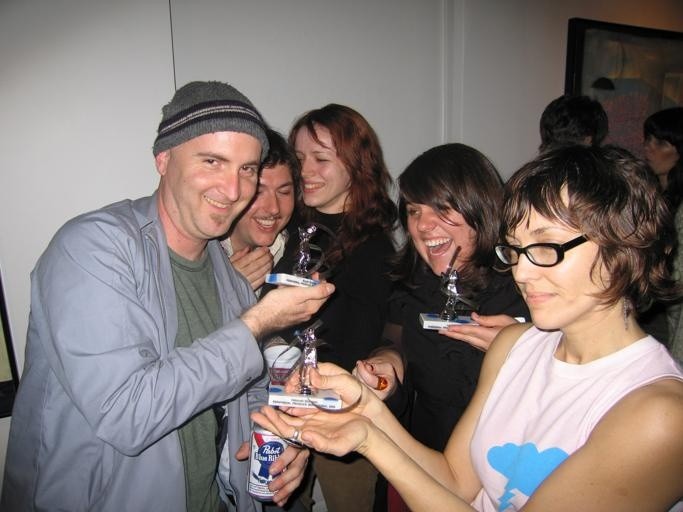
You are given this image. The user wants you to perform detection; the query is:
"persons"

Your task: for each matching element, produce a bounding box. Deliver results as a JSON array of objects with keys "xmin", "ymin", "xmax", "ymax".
[
  {"xmin": 349, "ymin": 142, "xmax": 531, "ymax": 512},
  {"xmin": 256, "ymin": 101, "xmax": 400, "ymax": 512},
  {"xmin": 536, "ymin": 92, "xmax": 610, "ymax": 157},
  {"xmin": 247, "ymin": 138, "xmax": 683, "ymax": 512},
  {"xmin": 1, "ymin": 79, "xmax": 340, "ymax": 511},
  {"xmin": 218, "ymin": 128, "xmax": 298, "ymax": 512},
  {"xmin": 633, "ymin": 104, "xmax": 683, "ymax": 370}
]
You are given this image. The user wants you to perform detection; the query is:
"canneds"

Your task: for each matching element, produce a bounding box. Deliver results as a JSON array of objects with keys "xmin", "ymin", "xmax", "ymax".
[{"xmin": 247, "ymin": 422, "xmax": 288, "ymax": 502}]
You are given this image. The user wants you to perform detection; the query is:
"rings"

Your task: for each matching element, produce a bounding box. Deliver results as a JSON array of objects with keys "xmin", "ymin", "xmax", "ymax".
[
  {"xmin": 373, "ymin": 375, "xmax": 388, "ymax": 390},
  {"xmin": 283, "ymin": 429, "xmax": 303, "ymax": 449}
]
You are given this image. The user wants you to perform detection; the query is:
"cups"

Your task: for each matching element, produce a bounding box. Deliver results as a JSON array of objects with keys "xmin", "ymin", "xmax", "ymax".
[{"xmin": 263, "ymin": 346, "xmax": 303, "ymax": 382}]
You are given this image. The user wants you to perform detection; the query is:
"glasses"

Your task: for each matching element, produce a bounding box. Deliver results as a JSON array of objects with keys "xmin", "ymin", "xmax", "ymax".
[{"xmin": 493, "ymin": 229, "xmax": 604, "ymax": 267}]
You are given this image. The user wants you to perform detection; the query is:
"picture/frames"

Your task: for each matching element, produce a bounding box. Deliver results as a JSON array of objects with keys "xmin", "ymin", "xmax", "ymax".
[{"xmin": 563, "ymin": 16, "xmax": 682, "ymax": 163}]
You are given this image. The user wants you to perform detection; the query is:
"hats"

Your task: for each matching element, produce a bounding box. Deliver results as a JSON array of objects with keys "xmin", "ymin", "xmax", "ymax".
[{"xmin": 153, "ymin": 81, "xmax": 271, "ymax": 163}]
